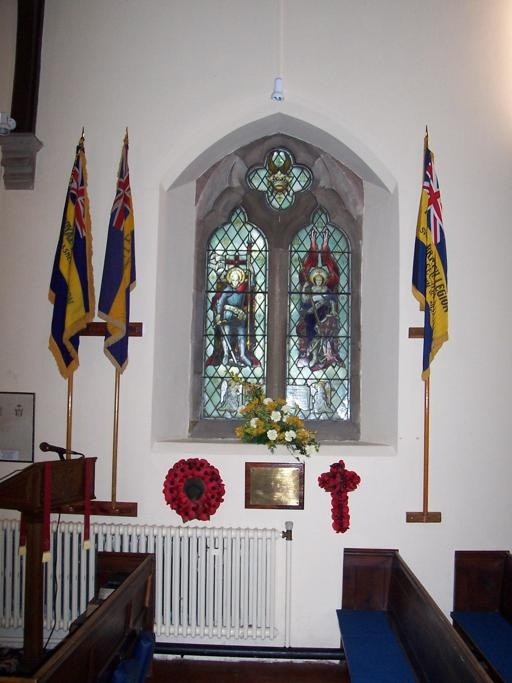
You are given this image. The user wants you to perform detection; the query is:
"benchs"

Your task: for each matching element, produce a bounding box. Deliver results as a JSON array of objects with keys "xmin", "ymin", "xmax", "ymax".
[
  {"xmin": 336, "ymin": 545, "xmax": 503, "ymax": 683},
  {"xmin": 0, "ymin": 551, "xmax": 157, "ymax": 683},
  {"xmin": 452, "ymin": 549, "xmax": 512, "ymax": 683}
]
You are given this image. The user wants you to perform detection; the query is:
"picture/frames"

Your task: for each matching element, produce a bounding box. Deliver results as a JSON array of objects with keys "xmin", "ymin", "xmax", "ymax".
[
  {"xmin": 245, "ymin": 462, "xmax": 305, "ymax": 509},
  {"xmin": 0, "ymin": 391, "xmax": 35, "ymax": 463}
]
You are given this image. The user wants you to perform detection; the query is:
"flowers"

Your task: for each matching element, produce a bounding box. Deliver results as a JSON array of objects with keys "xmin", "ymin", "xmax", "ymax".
[{"xmin": 233, "ymin": 374, "xmax": 321, "ymax": 460}]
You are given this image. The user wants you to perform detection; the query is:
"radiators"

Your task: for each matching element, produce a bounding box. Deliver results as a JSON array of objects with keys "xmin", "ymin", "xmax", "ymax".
[{"xmin": 0, "ymin": 517, "xmax": 293, "ymax": 652}]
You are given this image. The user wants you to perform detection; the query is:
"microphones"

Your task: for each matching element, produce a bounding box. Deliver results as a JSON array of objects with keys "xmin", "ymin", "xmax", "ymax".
[{"xmin": 38, "ymin": 440, "xmax": 83, "ymax": 455}]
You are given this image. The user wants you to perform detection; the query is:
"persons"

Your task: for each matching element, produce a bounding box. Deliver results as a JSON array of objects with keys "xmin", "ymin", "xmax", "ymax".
[
  {"xmin": 211, "ymin": 268, "xmax": 258, "ymax": 367},
  {"xmin": 300, "ymin": 265, "xmax": 345, "ymax": 367}
]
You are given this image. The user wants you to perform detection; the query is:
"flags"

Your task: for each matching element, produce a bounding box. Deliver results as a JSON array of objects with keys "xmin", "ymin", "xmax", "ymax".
[
  {"xmin": 411, "ymin": 130, "xmax": 457, "ymax": 381},
  {"xmin": 90, "ymin": 128, "xmax": 138, "ymax": 374},
  {"xmin": 45, "ymin": 127, "xmax": 102, "ymax": 379}
]
